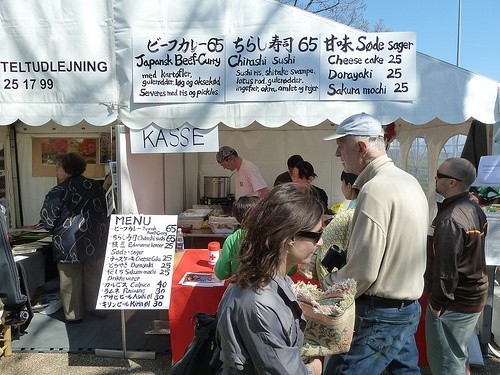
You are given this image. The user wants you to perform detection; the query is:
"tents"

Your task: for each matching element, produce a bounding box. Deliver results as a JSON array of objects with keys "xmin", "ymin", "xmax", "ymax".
[{"xmin": 0, "ymin": 0, "xmax": 500, "ymax": 347}]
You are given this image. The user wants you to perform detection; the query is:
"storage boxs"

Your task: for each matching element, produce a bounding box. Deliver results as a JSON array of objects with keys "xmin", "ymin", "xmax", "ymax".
[{"xmin": 180, "ymin": 201, "xmax": 240, "ymax": 233}]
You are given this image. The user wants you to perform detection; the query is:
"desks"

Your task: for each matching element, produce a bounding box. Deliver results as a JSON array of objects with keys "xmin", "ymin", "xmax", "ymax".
[
  {"xmin": 0, "ymin": 225, "xmax": 62, "ymax": 304},
  {"xmin": 170, "ymin": 248, "xmax": 323, "ymax": 366},
  {"xmin": 182, "ymin": 233, "xmax": 227, "ymax": 249}
]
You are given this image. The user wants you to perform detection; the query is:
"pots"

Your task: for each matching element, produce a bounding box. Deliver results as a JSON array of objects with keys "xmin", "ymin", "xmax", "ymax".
[{"xmin": 204, "ymin": 175, "xmax": 231, "ymax": 198}]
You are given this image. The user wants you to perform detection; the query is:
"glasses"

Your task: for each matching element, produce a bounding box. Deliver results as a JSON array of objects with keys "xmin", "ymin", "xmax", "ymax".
[
  {"xmin": 297, "ymin": 227, "xmax": 324, "ymax": 243},
  {"xmin": 437, "ymin": 169, "xmax": 462, "ymax": 182}
]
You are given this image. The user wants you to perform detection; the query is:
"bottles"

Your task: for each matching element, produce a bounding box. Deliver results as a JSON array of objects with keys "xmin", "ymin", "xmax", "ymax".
[{"xmin": 176, "ymin": 228, "xmax": 184, "ymax": 253}]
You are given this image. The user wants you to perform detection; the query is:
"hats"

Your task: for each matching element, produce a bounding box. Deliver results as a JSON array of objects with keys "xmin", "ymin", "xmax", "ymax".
[{"xmin": 322, "ymin": 112, "xmax": 385, "ymax": 141}]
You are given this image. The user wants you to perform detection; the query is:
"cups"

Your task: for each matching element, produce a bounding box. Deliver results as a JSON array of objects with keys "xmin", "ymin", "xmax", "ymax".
[{"xmin": 208, "ymin": 242, "xmax": 220, "ymax": 269}]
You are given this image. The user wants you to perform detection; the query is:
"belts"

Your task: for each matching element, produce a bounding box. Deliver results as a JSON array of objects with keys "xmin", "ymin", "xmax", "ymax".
[{"xmin": 355, "ymin": 292, "xmax": 415, "ymax": 309}]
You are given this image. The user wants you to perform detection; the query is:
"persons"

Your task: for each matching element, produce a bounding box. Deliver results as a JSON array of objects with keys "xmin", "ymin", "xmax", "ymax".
[
  {"xmin": 40, "ymin": 152, "xmax": 111, "ymax": 322},
  {"xmin": 214, "ymin": 182, "xmax": 325, "ymax": 375},
  {"xmin": 300, "ymin": 171, "xmax": 361, "ymax": 364},
  {"xmin": 323, "ymin": 113, "xmax": 429, "ymax": 375},
  {"xmin": 215, "ymin": 144, "xmax": 329, "ymax": 286},
  {"xmin": 426, "ymin": 157, "xmax": 489, "ymax": 375}
]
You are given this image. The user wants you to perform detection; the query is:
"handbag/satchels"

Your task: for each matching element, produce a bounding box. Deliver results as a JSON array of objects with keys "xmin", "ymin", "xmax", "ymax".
[{"xmin": 169, "ymin": 312, "xmax": 223, "ymax": 375}]
